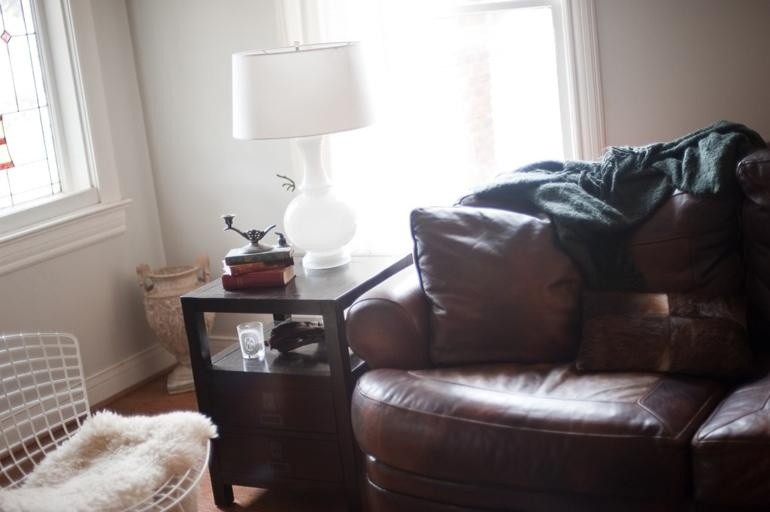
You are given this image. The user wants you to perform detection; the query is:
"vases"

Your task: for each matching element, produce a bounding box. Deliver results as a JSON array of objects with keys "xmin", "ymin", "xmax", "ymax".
[{"xmin": 134, "ymin": 255, "xmax": 215, "ymax": 394}]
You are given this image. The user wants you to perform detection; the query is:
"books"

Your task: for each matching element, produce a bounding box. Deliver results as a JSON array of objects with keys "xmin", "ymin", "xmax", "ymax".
[
  {"xmin": 219, "ymin": 258, "xmax": 295, "ymax": 277},
  {"xmin": 221, "ymin": 244, "xmax": 295, "ymax": 265},
  {"xmin": 220, "ymin": 265, "xmax": 297, "ymax": 291}
]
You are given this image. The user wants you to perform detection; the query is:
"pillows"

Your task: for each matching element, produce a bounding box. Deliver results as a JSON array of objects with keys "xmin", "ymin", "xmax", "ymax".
[
  {"xmin": 410, "ymin": 201, "xmax": 582, "ymax": 368},
  {"xmin": 575, "ymin": 290, "xmax": 748, "ymax": 378}
]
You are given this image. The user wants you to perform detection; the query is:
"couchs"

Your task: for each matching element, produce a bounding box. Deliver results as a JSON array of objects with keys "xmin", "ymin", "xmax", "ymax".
[{"xmin": 344, "ymin": 123, "xmax": 768, "ymax": 509}]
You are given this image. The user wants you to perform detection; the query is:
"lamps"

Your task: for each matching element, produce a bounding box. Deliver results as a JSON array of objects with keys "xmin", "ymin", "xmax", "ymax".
[{"xmin": 232, "ymin": 41, "xmax": 372, "ymax": 270}]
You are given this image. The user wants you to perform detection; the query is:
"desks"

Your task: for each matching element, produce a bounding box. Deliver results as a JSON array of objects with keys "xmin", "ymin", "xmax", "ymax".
[{"xmin": 180, "ymin": 250, "xmax": 417, "ymax": 511}]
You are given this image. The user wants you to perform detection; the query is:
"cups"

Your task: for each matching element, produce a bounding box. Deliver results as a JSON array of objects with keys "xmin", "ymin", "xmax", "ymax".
[{"xmin": 235, "ymin": 321, "xmax": 266, "ymax": 360}]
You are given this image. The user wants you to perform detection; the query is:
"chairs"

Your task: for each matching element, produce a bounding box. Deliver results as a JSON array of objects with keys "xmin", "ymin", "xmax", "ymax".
[{"xmin": 0, "ymin": 327, "xmax": 214, "ymax": 510}]
